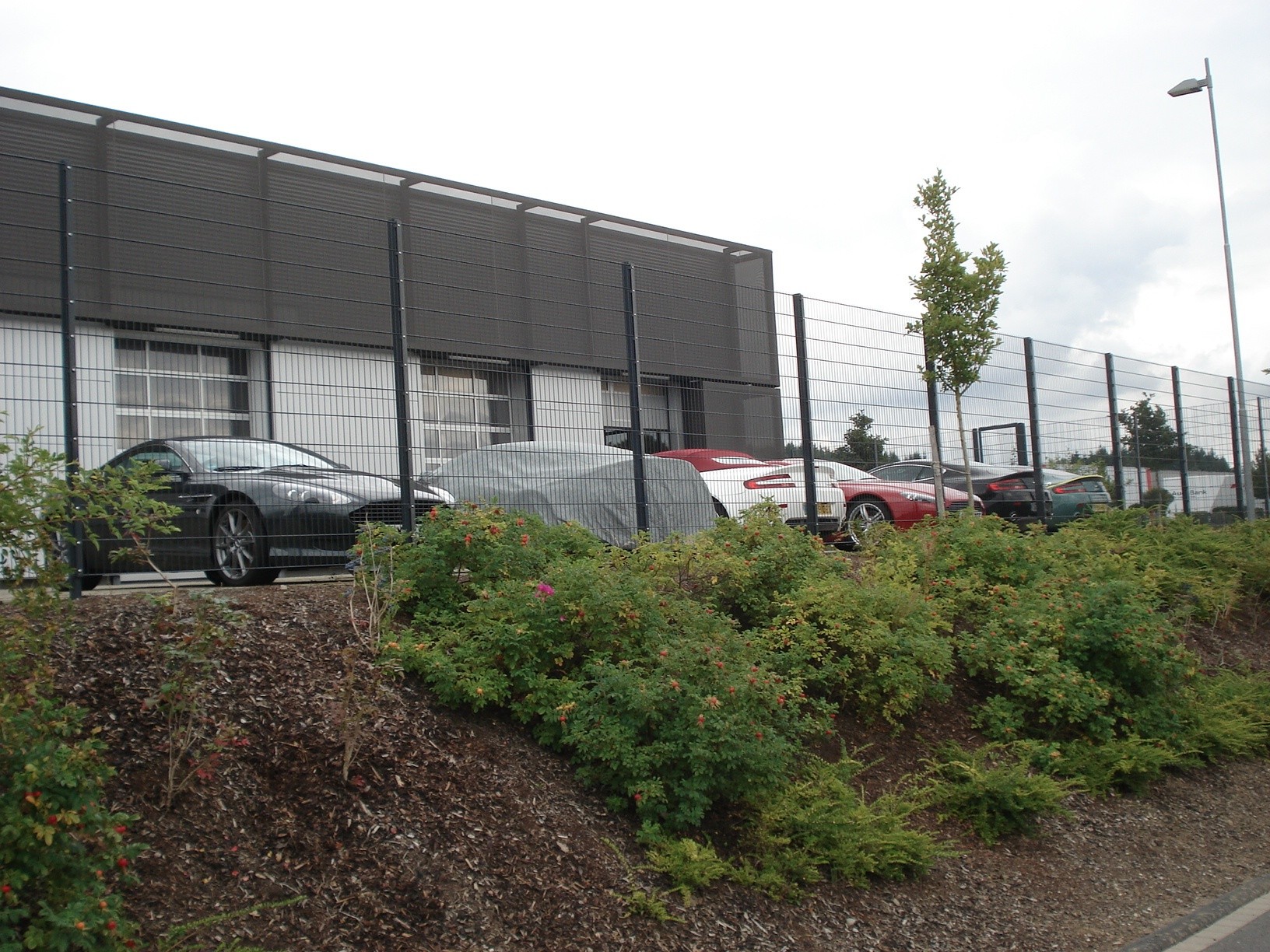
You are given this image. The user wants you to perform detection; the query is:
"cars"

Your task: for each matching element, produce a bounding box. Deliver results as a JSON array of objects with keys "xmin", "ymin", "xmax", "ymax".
[
  {"xmin": 866, "ymin": 458, "xmax": 1053, "ymax": 536},
  {"xmin": 40, "ymin": 435, "xmax": 458, "ymax": 586},
  {"xmin": 1043, "ymin": 465, "xmax": 1114, "ymax": 534},
  {"xmin": 764, "ymin": 457, "xmax": 987, "ymax": 549},
  {"xmin": 650, "ymin": 449, "xmax": 847, "ymax": 538},
  {"xmin": 420, "ymin": 439, "xmax": 718, "ymax": 549}
]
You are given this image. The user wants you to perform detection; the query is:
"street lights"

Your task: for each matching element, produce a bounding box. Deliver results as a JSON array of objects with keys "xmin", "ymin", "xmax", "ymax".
[{"xmin": 1167, "ymin": 56, "xmax": 1256, "ymax": 523}]
